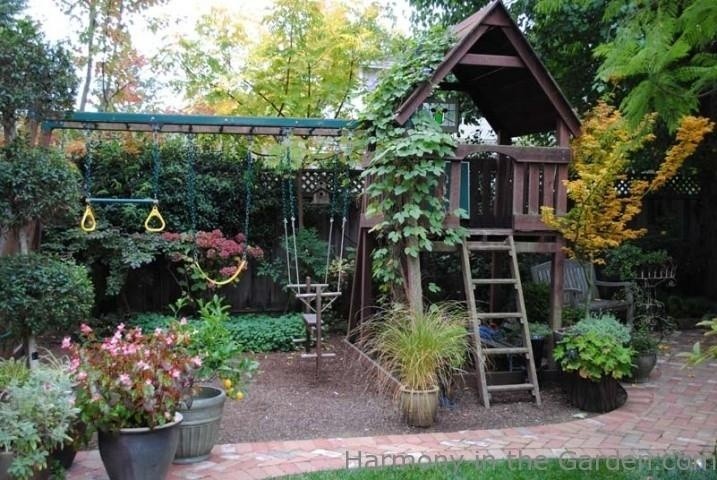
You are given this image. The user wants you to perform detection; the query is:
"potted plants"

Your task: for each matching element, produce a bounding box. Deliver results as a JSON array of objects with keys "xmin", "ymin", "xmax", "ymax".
[
  {"xmin": 524, "ymin": 310, "xmax": 663, "ymax": 411},
  {"xmin": 367, "ymin": 301, "xmax": 471, "ymax": 429}
]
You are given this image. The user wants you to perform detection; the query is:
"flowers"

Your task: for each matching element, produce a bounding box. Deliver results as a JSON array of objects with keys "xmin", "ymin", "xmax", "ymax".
[
  {"xmin": 164, "ymin": 229, "xmax": 263, "ymax": 298},
  {"xmin": 40, "ymin": 306, "xmax": 254, "ymax": 430}
]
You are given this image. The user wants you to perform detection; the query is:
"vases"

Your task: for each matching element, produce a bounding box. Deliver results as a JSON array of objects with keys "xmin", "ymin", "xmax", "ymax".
[{"xmin": 99, "ymin": 387, "xmax": 224, "ymax": 480}]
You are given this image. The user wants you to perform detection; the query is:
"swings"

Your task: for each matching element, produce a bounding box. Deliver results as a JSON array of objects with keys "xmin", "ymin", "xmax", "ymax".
[
  {"xmin": 186, "ymin": 133, "xmax": 252, "ymax": 284},
  {"xmin": 279, "ymin": 128, "xmax": 351, "ymax": 327}
]
